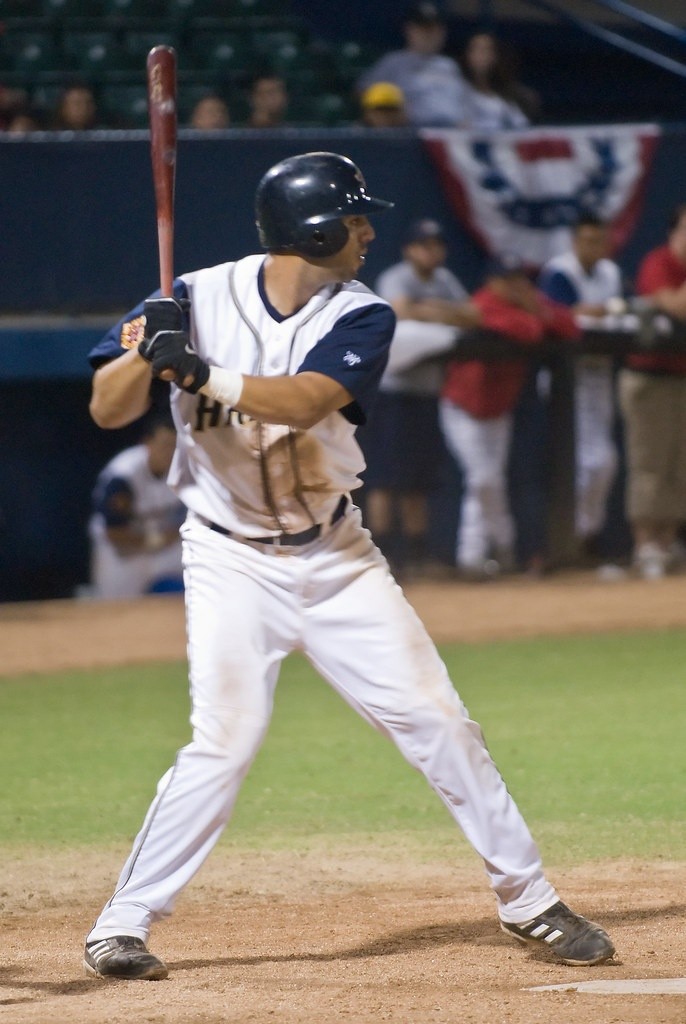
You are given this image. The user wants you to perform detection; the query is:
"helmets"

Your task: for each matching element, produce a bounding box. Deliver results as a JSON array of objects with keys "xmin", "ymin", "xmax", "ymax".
[{"xmin": 254, "ymin": 152, "xmax": 394, "ymax": 258}]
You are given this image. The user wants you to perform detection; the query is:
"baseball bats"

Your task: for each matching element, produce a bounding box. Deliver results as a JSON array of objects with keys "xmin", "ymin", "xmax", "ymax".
[{"xmin": 146, "ymin": 42, "xmax": 181, "ymax": 377}]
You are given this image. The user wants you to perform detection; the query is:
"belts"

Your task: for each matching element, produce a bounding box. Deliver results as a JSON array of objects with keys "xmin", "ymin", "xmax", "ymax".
[{"xmin": 211, "ymin": 495, "xmax": 347, "ymax": 546}]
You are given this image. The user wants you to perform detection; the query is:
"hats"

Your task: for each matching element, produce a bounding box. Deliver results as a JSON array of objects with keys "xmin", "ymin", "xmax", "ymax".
[
  {"xmin": 399, "ymin": 218, "xmax": 445, "ymax": 244},
  {"xmin": 363, "ymin": 82, "xmax": 403, "ymax": 108}
]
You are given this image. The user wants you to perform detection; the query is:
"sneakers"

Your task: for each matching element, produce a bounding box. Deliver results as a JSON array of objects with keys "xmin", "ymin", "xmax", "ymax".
[
  {"xmin": 498, "ymin": 899, "xmax": 616, "ymax": 965},
  {"xmin": 84, "ymin": 935, "xmax": 168, "ymax": 981}
]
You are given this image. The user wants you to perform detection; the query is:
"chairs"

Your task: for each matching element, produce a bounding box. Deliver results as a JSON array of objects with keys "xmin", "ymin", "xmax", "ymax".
[{"xmin": 0, "ymin": 0, "xmax": 376, "ymax": 129}]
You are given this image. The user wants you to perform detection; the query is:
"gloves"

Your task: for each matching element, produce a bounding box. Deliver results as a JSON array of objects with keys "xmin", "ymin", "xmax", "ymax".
[
  {"xmin": 138, "ymin": 298, "xmax": 191, "ymax": 362},
  {"xmin": 144, "ymin": 330, "xmax": 210, "ymax": 395}
]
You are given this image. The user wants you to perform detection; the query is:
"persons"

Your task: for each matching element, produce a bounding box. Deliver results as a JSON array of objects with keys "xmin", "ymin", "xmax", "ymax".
[
  {"xmin": 71, "ymin": 415, "xmax": 190, "ymax": 600},
  {"xmin": 241, "ymin": 72, "xmax": 289, "ymax": 127},
  {"xmin": 188, "ymin": 95, "xmax": 233, "ymax": 131},
  {"xmin": 358, "ymin": 216, "xmax": 686, "ymax": 573},
  {"xmin": 349, "ymin": 0, "xmax": 541, "ymax": 129},
  {"xmin": 0, "ymin": 77, "xmax": 102, "ymax": 131},
  {"xmin": 88, "ymin": 153, "xmax": 612, "ymax": 980}
]
